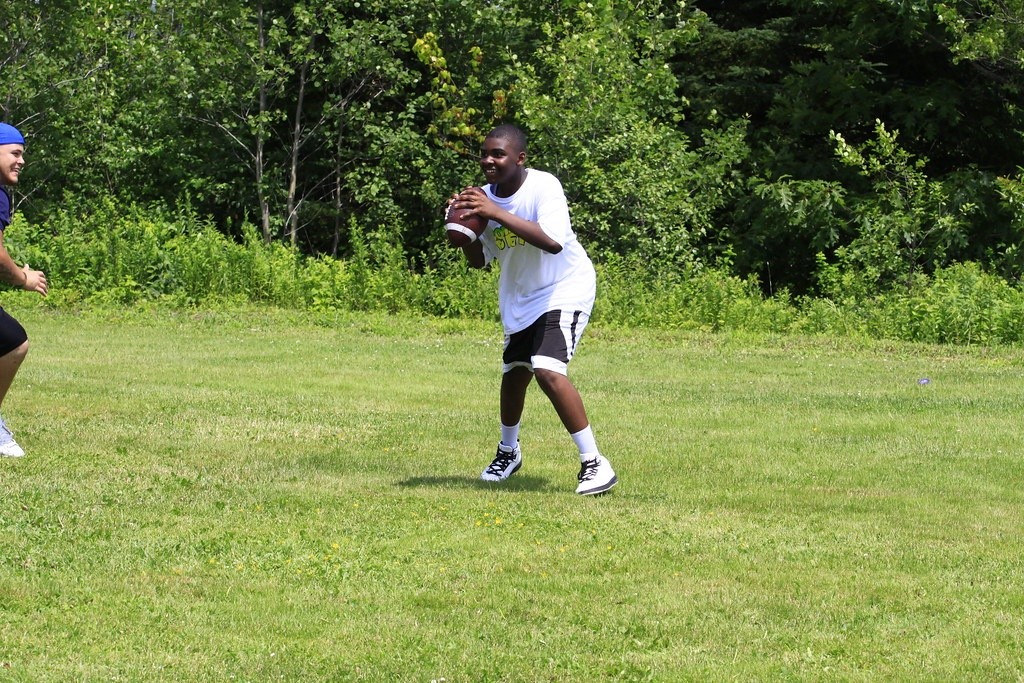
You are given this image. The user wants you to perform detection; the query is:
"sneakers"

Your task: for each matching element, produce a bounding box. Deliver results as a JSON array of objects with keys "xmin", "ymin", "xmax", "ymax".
[
  {"xmin": 575, "ymin": 453, "xmax": 619, "ymax": 496},
  {"xmin": 0, "ymin": 416, "xmax": 25, "ymax": 457},
  {"xmin": 480, "ymin": 438, "xmax": 522, "ymax": 482}
]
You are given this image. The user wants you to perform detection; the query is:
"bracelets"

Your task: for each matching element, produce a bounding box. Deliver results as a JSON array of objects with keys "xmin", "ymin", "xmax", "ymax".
[{"xmin": 16, "ymin": 272, "xmax": 28, "ymax": 287}]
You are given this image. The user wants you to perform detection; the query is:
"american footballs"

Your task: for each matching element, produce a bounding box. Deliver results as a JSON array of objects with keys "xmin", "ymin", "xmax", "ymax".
[{"xmin": 445, "ymin": 187, "xmax": 489, "ymax": 247}]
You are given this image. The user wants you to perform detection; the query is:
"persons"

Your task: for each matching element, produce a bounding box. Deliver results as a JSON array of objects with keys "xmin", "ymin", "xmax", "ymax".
[
  {"xmin": 443, "ymin": 125, "xmax": 619, "ymax": 496},
  {"xmin": 0, "ymin": 121, "xmax": 48, "ymax": 459}
]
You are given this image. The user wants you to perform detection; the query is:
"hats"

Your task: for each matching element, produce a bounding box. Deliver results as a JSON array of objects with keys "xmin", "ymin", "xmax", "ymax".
[{"xmin": 0, "ymin": 122, "xmax": 25, "ymax": 144}]
